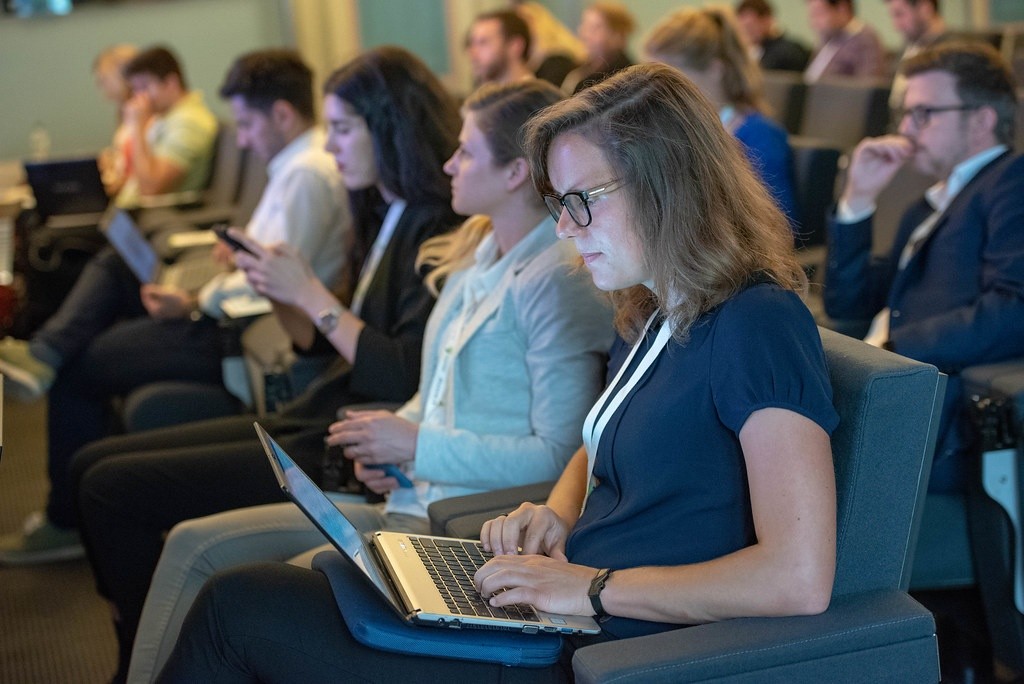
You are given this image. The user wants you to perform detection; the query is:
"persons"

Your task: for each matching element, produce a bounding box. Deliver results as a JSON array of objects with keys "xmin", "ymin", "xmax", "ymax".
[
  {"xmin": 129, "ymin": 76, "xmax": 612, "ymax": 684},
  {"xmin": 806, "ymin": 0, "xmax": 887, "ymax": 79},
  {"xmin": 0, "ymin": 47, "xmax": 354, "ymax": 566},
  {"xmin": 65, "ymin": 44, "xmax": 468, "ymax": 684},
  {"xmin": 824, "ymin": 40, "xmax": 1024, "ymax": 491},
  {"xmin": 465, "ymin": 0, "xmax": 639, "ymax": 96},
  {"xmin": 638, "ymin": 1, "xmax": 794, "ymax": 225},
  {"xmin": 0, "ymin": 38, "xmax": 217, "ymax": 397},
  {"xmin": 885, "ymin": 0, "xmax": 948, "ymax": 57},
  {"xmin": 736, "ymin": 1, "xmax": 811, "ymax": 72}
]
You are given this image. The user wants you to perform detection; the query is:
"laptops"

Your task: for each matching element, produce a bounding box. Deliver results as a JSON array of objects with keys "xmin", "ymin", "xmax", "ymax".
[
  {"xmin": 254, "ymin": 421, "xmax": 602, "ymax": 636},
  {"xmin": 26, "ymin": 157, "xmax": 110, "ymax": 228},
  {"xmin": 98, "ymin": 202, "xmax": 232, "ymax": 295}
]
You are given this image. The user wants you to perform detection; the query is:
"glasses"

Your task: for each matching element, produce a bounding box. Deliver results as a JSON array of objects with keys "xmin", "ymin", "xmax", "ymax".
[
  {"xmin": 890, "ymin": 103, "xmax": 977, "ymax": 129},
  {"xmin": 540, "ymin": 175, "xmax": 630, "ymax": 228}
]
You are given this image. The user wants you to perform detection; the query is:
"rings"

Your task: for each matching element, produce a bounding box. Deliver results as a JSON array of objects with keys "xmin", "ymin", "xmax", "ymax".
[{"xmin": 496, "ymin": 512, "xmax": 508, "ymax": 517}]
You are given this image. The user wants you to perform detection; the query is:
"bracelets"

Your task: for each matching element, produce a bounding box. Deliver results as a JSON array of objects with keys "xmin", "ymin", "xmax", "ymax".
[{"xmin": 588, "ymin": 566, "xmax": 613, "ymax": 617}]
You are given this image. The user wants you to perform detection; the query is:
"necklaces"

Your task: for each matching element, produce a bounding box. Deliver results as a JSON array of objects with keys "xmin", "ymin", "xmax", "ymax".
[{"xmin": 153, "ymin": 61, "xmax": 842, "ymax": 684}]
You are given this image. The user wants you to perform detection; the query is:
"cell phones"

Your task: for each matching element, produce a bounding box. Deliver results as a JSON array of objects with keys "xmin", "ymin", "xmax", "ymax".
[{"xmin": 211, "ymin": 223, "xmax": 253, "ymax": 255}]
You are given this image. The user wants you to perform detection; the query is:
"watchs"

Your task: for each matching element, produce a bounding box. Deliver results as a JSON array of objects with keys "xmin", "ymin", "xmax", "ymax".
[{"xmin": 314, "ymin": 305, "xmax": 345, "ymax": 334}]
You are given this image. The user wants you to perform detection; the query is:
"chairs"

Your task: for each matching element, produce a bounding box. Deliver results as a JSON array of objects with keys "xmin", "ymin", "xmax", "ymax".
[{"xmin": 148, "ymin": 120, "xmax": 1023, "ymax": 684}]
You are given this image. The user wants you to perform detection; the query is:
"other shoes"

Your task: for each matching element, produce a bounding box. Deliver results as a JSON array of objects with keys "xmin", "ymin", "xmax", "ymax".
[
  {"xmin": 0, "ymin": 337, "xmax": 57, "ymax": 397},
  {"xmin": 0, "ymin": 511, "xmax": 86, "ymax": 564}
]
